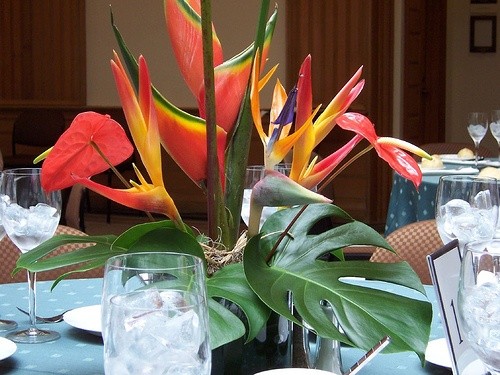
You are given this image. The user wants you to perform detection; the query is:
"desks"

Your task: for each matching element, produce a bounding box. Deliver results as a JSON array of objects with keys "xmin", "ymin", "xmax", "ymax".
[
  {"xmin": 0, "ymin": 99, "xmax": 200, "ymax": 223},
  {"xmin": 384, "ymin": 156, "xmax": 500, "ymax": 236},
  {"xmin": 0, "ymin": 277, "xmax": 447, "ymax": 375}
]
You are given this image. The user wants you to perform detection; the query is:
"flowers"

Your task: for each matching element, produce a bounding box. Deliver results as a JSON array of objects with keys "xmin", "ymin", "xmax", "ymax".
[{"xmin": 11, "ymin": 0, "xmax": 434, "ymax": 368}]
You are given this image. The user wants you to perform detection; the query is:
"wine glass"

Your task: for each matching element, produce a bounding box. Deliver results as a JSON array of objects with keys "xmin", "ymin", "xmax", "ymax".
[
  {"xmin": 465, "ymin": 112, "xmax": 488, "ymax": 170},
  {"xmin": 0, "ymin": 168, "xmax": 63, "ymax": 343}
]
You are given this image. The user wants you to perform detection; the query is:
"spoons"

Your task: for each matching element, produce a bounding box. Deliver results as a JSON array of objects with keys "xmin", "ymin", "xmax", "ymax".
[{"xmin": 0, "ymin": 320, "xmax": 18, "ymax": 334}]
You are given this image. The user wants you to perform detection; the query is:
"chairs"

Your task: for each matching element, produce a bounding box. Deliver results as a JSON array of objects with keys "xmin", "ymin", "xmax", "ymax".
[
  {"xmin": 5, "ymin": 108, "xmax": 66, "ymax": 170},
  {"xmin": 0, "ymin": 223, "xmax": 105, "ymax": 285},
  {"xmin": 96, "ymin": 152, "xmax": 138, "ymax": 224},
  {"xmin": 391, "ymin": 138, "xmax": 490, "ymax": 184},
  {"xmin": 369, "ymin": 219, "xmax": 446, "ymax": 285},
  {"xmin": 64, "ymin": 173, "xmax": 90, "ymax": 234}
]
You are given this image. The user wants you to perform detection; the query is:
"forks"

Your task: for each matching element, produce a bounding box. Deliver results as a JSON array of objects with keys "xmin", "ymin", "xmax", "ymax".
[{"xmin": 15, "ymin": 306, "xmax": 73, "ymax": 322}]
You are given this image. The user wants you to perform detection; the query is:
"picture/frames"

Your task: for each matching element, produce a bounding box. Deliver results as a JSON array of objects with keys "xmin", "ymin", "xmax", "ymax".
[{"xmin": 470, "ymin": 16, "xmax": 496, "ymax": 53}]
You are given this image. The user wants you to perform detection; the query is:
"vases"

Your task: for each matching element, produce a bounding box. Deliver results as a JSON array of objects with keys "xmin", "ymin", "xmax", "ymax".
[{"xmin": 208, "ymin": 289, "xmax": 293, "ymax": 375}]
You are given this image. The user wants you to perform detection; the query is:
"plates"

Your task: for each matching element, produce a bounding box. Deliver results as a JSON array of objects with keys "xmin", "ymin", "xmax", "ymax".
[
  {"xmin": 425, "ymin": 337, "xmax": 451, "ymax": 368},
  {"xmin": 62, "ymin": 304, "xmax": 102, "ymax": 336},
  {"xmin": 435, "ymin": 153, "xmax": 484, "ymax": 160},
  {"xmin": 0, "ymin": 337, "xmax": 17, "ymax": 361},
  {"xmin": 417, "ymin": 168, "xmax": 479, "ymax": 174}
]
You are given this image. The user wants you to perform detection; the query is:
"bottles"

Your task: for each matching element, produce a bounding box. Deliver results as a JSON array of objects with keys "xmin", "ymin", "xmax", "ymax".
[{"xmin": 288, "ymin": 300, "xmax": 343, "ymax": 375}]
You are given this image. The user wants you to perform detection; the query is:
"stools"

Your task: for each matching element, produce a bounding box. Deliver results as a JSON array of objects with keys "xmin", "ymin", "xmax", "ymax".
[{"xmin": 334, "ymin": 236, "xmax": 383, "ymax": 263}]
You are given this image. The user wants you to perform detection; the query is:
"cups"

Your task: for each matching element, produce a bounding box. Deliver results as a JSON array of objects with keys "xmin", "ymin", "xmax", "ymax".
[
  {"xmin": 488, "ymin": 110, "xmax": 500, "ymax": 146},
  {"xmin": 240, "ymin": 164, "xmax": 318, "ymax": 233},
  {"xmin": 100, "ymin": 251, "xmax": 213, "ymax": 375},
  {"xmin": 252, "ymin": 367, "xmax": 336, "ymax": 375},
  {"xmin": 435, "ymin": 173, "xmax": 500, "ymax": 256},
  {"xmin": 458, "ymin": 238, "xmax": 500, "ymax": 374}
]
